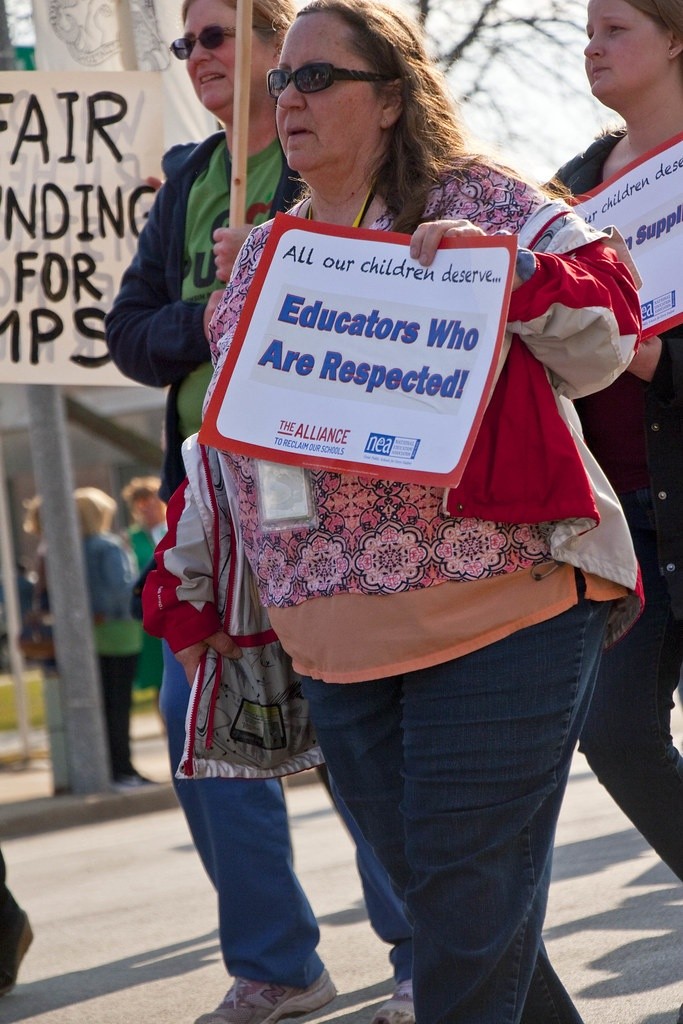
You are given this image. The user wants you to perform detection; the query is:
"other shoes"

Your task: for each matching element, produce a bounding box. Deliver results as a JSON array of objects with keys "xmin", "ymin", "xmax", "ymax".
[
  {"xmin": 0, "ymin": 909, "xmax": 34, "ymax": 996},
  {"xmin": 116, "ymin": 770, "xmax": 153, "ymax": 792}
]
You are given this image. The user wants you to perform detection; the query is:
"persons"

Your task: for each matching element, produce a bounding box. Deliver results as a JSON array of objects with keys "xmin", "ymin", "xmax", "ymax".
[
  {"xmin": 142, "ymin": 0, "xmax": 651, "ymax": 1024},
  {"xmin": 534, "ymin": 0, "xmax": 683, "ymax": 887},
  {"xmin": 104, "ymin": 0, "xmax": 416, "ymax": 1022},
  {"xmin": 28, "ymin": 476, "xmax": 169, "ymax": 786}
]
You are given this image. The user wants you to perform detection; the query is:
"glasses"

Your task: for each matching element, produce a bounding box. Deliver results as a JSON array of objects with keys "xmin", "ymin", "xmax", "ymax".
[
  {"xmin": 168, "ymin": 25, "xmax": 224, "ymax": 60},
  {"xmin": 266, "ymin": 62, "xmax": 382, "ymax": 98}
]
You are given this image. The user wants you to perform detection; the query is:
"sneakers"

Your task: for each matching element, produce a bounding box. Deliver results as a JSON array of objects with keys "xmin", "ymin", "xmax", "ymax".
[
  {"xmin": 193, "ymin": 960, "xmax": 337, "ymax": 1024},
  {"xmin": 370, "ymin": 979, "xmax": 417, "ymax": 1024}
]
